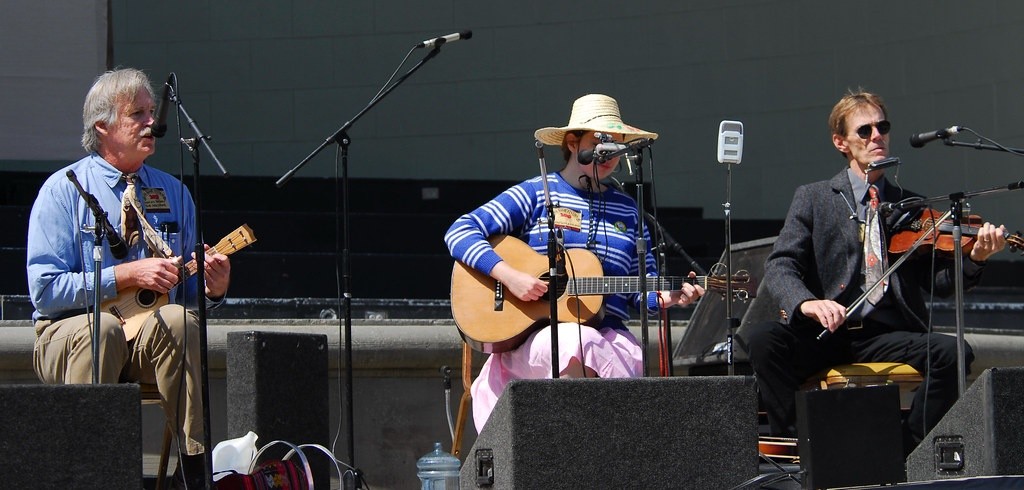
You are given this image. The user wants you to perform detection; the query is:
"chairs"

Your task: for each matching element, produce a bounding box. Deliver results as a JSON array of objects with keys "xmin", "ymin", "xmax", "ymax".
[
  {"xmin": 141, "ymin": 383, "xmax": 184, "ymax": 490},
  {"xmin": 451, "ymin": 342, "xmax": 473, "ymax": 459}
]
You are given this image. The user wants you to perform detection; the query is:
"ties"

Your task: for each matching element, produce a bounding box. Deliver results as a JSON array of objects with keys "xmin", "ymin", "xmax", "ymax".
[
  {"xmin": 865, "ymin": 186, "xmax": 884, "ymax": 306},
  {"xmin": 120, "ymin": 173, "xmax": 183, "ymax": 269}
]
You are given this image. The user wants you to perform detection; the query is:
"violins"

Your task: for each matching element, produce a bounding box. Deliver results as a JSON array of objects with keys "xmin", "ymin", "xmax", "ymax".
[{"xmin": 888, "ymin": 207, "xmax": 1024, "ymax": 261}]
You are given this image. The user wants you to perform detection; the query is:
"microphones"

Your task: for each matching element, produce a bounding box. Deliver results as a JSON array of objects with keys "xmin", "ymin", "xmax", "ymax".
[
  {"xmin": 910, "ymin": 125, "xmax": 965, "ymax": 148},
  {"xmin": 578, "ymin": 150, "xmax": 597, "ymax": 166},
  {"xmin": 880, "ymin": 202, "xmax": 896, "ymax": 217},
  {"xmin": 417, "ymin": 30, "xmax": 473, "ymax": 48},
  {"xmin": 151, "ymin": 73, "xmax": 174, "ymax": 138},
  {"xmin": 103, "ymin": 214, "xmax": 129, "ymax": 259}
]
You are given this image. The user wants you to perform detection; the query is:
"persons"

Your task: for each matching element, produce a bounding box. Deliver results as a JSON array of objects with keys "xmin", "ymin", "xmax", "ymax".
[
  {"xmin": 443, "ymin": 93, "xmax": 706, "ymax": 435},
  {"xmin": 27, "ymin": 66, "xmax": 230, "ymax": 490},
  {"xmin": 747, "ymin": 87, "xmax": 1006, "ymax": 462}
]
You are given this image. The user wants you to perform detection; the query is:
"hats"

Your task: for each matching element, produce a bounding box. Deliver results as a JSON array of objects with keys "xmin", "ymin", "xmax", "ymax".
[{"xmin": 533, "ymin": 94, "xmax": 658, "ymax": 145}]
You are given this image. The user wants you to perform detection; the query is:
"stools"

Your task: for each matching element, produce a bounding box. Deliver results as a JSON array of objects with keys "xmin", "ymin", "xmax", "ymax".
[{"xmin": 799, "ymin": 359, "xmax": 924, "ymax": 391}]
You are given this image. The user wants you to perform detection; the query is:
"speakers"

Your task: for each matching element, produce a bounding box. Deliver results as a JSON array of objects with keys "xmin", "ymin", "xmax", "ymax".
[
  {"xmin": 0, "ymin": 382, "xmax": 147, "ymax": 490},
  {"xmin": 795, "ymin": 383, "xmax": 905, "ymax": 490},
  {"xmin": 672, "ymin": 235, "xmax": 784, "ymax": 367},
  {"xmin": 226, "ymin": 332, "xmax": 332, "ymax": 490},
  {"xmin": 906, "ymin": 365, "xmax": 1024, "ymax": 485},
  {"xmin": 460, "ymin": 375, "xmax": 761, "ymax": 490}
]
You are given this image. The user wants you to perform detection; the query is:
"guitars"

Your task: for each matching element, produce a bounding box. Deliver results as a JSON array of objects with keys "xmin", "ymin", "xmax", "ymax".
[
  {"xmin": 100, "ymin": 222, "xmax": 258, "ymax": 342},
  {"xmin": 450, "ymin": 234, "xmax": 758, "ymax": 354}
]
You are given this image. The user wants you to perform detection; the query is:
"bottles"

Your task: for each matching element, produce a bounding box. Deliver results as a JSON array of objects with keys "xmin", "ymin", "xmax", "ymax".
[
  {"xmin": 212, "ymin": 431, "xmax": 259, "ymax": 482},
  {"xmin": 416, "ymin": 442, "xmax": 461, "ymax": 490}
]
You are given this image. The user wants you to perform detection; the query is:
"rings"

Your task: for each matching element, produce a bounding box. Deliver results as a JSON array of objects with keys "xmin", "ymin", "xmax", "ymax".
[{"xmin": 984, "ymin": 239, "xmax": 990, "ymax": 242}]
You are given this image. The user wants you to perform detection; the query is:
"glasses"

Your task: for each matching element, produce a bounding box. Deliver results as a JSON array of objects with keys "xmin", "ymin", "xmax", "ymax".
[{"xmin": 842, "ymin": 119, "xmax": 892, "ymax": 139}]
